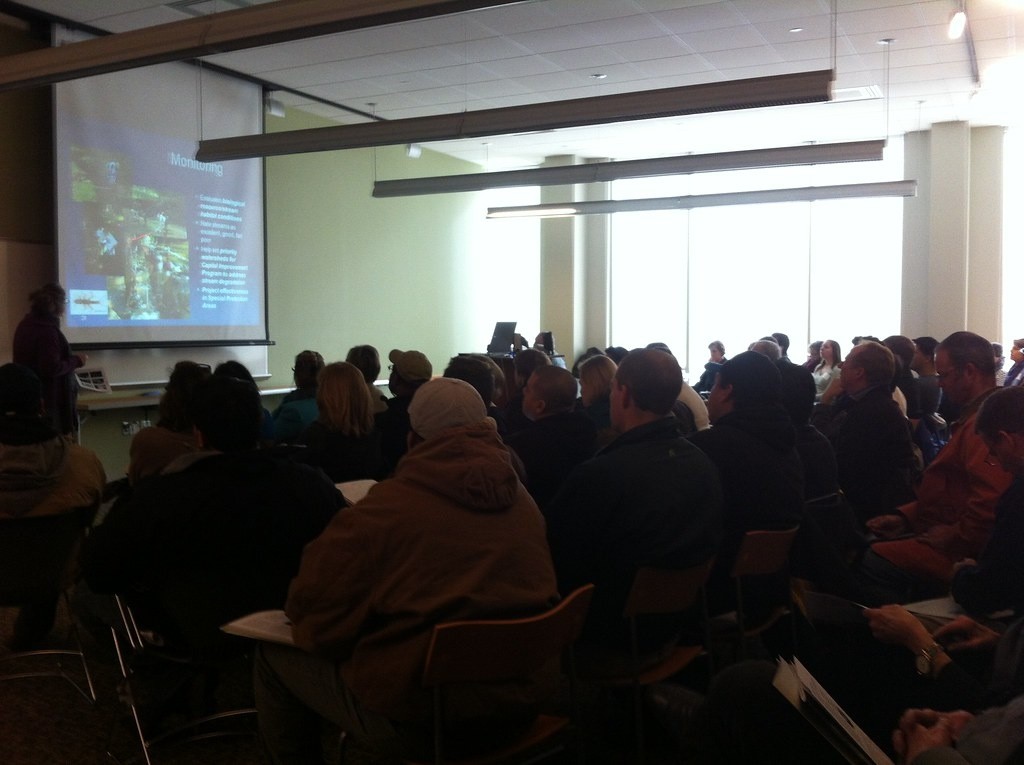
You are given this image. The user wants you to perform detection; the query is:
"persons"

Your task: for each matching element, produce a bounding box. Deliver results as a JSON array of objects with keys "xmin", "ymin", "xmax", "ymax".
[
  {"xmin": 13, "ymin": 282, "xmax": 85, "ymax": 445},
  {"xmin": 0, "ymin": 363, "xmax": 108, "ymax": 655},
  {"xmin": 71, "ymin": 333, "xmax": 1024, "ymax": 765}
]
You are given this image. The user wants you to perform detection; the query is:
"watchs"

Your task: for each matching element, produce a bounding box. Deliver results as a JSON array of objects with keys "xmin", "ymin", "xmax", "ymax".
[{"xmin": 915, "ymin": 644, "xmax": 943, "ymax": 679}]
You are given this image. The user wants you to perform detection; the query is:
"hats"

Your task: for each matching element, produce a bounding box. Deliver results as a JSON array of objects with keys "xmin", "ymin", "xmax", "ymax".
[
  {"xmin": 407, "ymin": 377, "xmax": 488, "ymax": 443},
  {"xmin": 388, "ymin": 349, "xmax": 433, "ymax": 384}
]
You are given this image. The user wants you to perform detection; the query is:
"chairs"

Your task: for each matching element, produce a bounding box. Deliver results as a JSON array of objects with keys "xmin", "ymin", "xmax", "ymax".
[{"xmin": 0, "ymin": 408, "xmax": 947, "ymax": 765}]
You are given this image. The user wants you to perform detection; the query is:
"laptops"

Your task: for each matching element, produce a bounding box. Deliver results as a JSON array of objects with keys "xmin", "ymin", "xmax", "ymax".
[{"xmin": 488, "ymin": 322, "xmax": 517, "ymax": 354}]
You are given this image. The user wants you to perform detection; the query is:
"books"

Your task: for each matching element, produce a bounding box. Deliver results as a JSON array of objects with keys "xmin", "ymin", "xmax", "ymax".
[{"xmin": 220, "ymin": 610, "xmax": 294, "ymax": 644}]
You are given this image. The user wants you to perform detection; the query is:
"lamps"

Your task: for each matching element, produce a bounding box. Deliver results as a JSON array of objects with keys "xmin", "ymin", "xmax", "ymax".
[
  {"xmin": 192, "ymin": 0, "xmax": 837, "ymax": 163},
  {"xmin": 263, "ymin": 87, "xmax": 286, "ymax": 117},
  {"xmin": 480, "ymin": 99, "xmax": 924, "ymax": 220},
  {"xmin": 0, "ymin": 0, "xmax": 527, "ymax": 98},
  {"xmin": 367, "ymin": 38, "xmax": 892, "ymax": 198}
]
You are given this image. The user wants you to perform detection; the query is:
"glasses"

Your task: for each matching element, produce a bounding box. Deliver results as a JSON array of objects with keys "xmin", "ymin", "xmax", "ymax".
[
  {"xmin": 389, "ymin": 364, "xmax": 397, "ymax": 375},
  {"xmin": 935, "ymin": 363, "xmax": 960, "ymax": 382},
  {"xmin": 63, "ymin": 296, "xmax": 70, "ymax": 305},
  {"xmin": 291, "ymin": 366, "xmax": 298, "ymax": 371}
]
